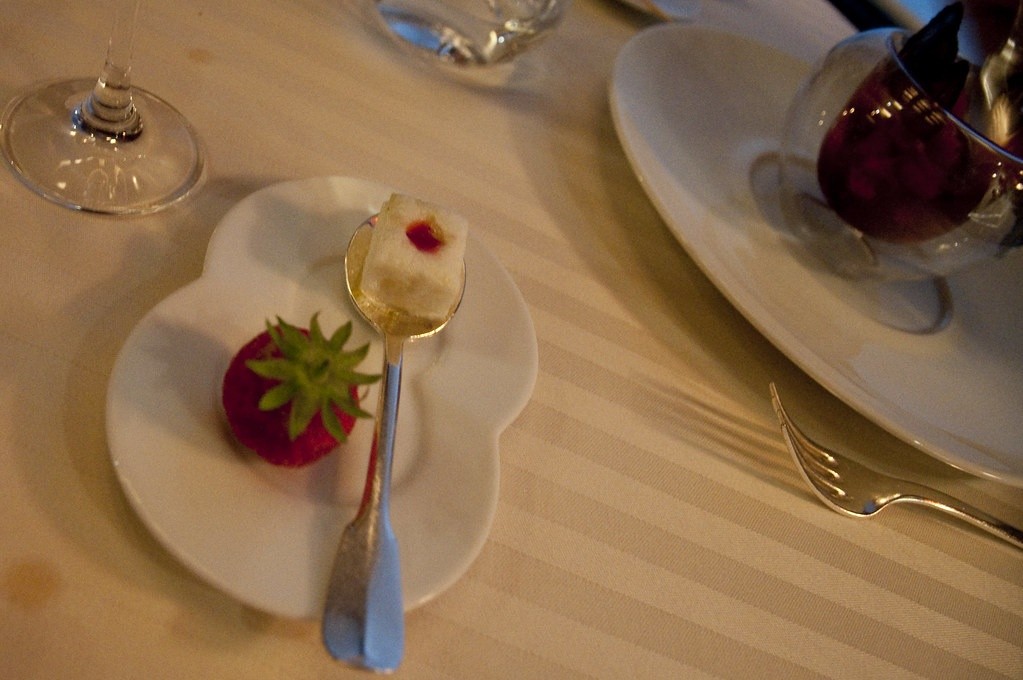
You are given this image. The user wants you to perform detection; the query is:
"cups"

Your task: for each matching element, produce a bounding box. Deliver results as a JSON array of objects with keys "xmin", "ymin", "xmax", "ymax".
[
  {"xmin": 779, "ymin": 0, "xmax": 1023, "ymax": 298},
  {"xmin": 374, "ymin": 0, "xmax": 563, "ymax": 70}
]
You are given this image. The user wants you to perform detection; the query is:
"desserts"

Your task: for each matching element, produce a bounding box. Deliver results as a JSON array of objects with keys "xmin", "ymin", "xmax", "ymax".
[{"xmin": 359, "ymin": 193, "xmax": 468, "ymax": 321}]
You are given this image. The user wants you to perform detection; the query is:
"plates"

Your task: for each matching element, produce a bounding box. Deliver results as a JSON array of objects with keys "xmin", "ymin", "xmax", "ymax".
[
  {"xmin": 606, "ymin": 20, "xmax": 1023, "ymax": 496},
  {"xmin": 104, "ymin": 174, "xmax": 538, "ymax": 624}
]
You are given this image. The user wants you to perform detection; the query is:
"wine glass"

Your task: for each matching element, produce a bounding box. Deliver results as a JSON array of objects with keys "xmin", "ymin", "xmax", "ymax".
[{"xmin": 0, "ymin": 0, "xmax": 206, "ymax": 219}]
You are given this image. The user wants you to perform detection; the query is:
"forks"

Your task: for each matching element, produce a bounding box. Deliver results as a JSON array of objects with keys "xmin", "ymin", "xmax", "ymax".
[{"xmin": 768, "ymin": 381, "xmax": 1023, "ymax": 554}]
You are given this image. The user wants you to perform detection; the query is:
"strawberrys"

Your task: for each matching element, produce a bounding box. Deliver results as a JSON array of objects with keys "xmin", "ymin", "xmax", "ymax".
[{"xmin": 221, "ymin": 323, "xmax": 359, "ymax": 468}]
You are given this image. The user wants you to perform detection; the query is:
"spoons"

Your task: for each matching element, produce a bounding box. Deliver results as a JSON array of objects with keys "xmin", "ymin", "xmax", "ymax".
[{"xmin": 320, "ymin": 213, "xmax": 467, "ymax": 674}]
